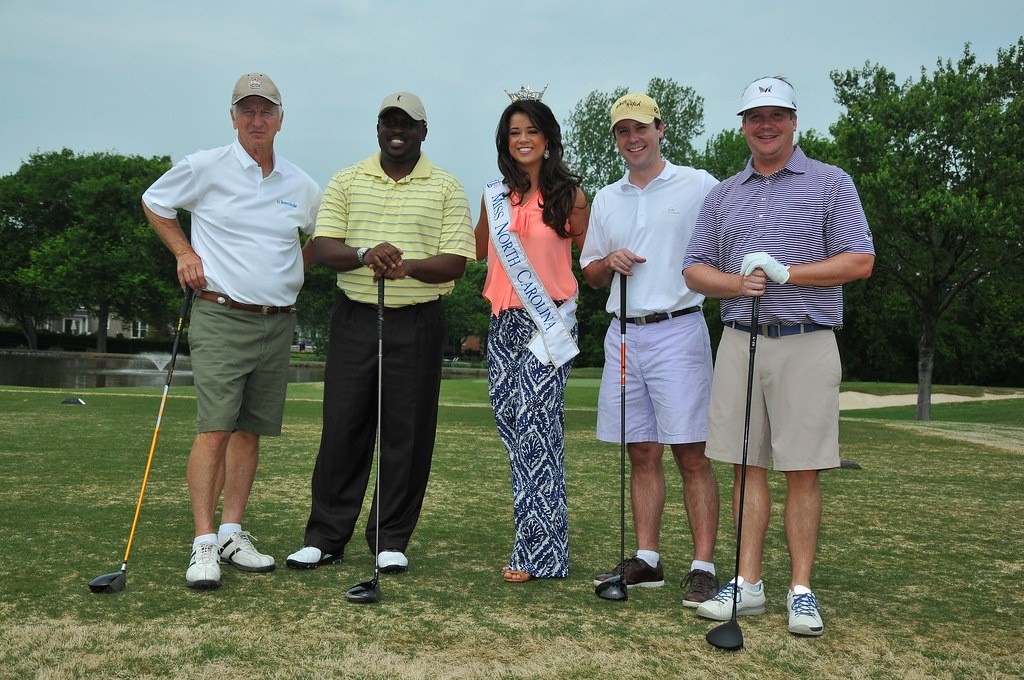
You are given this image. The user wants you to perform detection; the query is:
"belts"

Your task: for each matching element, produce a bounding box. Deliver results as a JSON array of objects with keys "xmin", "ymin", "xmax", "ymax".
[
  {"xmin": 725, "ymin": 314, "xmax": 832, "ymax": 339},
  {"xmin": 612, "ymin": 306, "xmax": 700, "ymax": 325},
  {"xmin": 197, "ymin": 288, "xmax": 298, "ymax": 316}
]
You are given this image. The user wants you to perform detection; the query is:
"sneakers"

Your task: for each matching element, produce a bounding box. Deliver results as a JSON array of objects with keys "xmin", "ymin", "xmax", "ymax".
[
  {"xmin": 785, "ymin": 587, "xmax": 823, "ymax": 636},
  {"xmin": 219, "ymin": 530, "xmax": 278, "ymax": 571},
  {"xmin": 680, "ymin": 566, "xmax": 718, "ymax": 608},
  {"xmin": 592, "ymin": 554, "xmax": 664, "ymax": 589},
  {"xmin": 186, "ymin": 540, "xmax": 222, "ymax": 587},
  {"xmin": 378, "ymin": 549, "xmax": 408, "ymax": 572},
  {"xmin": 287, "ymin": 542, "xmax": 344, "ymax": 567},
  {"xmin": 696, "ymin": 571, "xmax": 766, "ymax": 620}
]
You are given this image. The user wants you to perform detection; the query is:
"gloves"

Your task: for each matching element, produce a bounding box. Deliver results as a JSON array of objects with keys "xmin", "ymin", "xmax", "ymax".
[{"xmin": 740, "ymin": 253, "xmax": 791, "ymax": 287}]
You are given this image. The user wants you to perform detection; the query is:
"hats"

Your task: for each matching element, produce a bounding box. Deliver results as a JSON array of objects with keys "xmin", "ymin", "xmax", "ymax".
[
  {"xmin": 608, "ymin": 92, "xmax": 661, "ymax": 133},
  {"xmin": 377, "ymin": 92, "xmax": 427, "ymax": 125},
  {"xmin": 232, "ymin": 72, "xmax": 282, "ymax": 109},
  {"xmin": 736, "ymin": 77, "xmax": 796, "ymax": 117}
]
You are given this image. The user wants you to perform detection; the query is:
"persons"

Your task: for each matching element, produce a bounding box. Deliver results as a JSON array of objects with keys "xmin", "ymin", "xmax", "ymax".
[
  {"xmin": 580, "ymin": 94, "xmax": 722, "ymax": 607},
  {"xmin": 140, "ymin": 70, "xmax": 323, "ymax": 587},
  {"xmin": 681, "ymin": 76, "xmax": 876, "ymax": 637},
  {"xmin": 286, "ymin": 89, "xmax": 477, "ymax": 571},
  {"xmin": 474, "ymin": 82, "xmax": 589, "ymax": 582}
]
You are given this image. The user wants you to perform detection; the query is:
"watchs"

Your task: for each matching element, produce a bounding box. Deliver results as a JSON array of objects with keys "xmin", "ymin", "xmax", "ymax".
[{"xmin": 358, "ymin": 245, "xmax": 370, "ymax": 265}]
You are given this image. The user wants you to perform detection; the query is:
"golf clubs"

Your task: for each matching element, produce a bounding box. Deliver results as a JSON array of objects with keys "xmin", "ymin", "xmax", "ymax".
[
  {"xmin": 89, "ymin": 281, "xmax": 196, "ymax": 592},
  {"xmin": 346, "ymin": 273, "xmax": 386, "ymax": 605},
  {"xmin": 707, "ymin": 291, "xmax": 761, "ymax": 651},
  {"xmin": 595, "ymin": 273, "xmax": 629, "ymax": 600}
]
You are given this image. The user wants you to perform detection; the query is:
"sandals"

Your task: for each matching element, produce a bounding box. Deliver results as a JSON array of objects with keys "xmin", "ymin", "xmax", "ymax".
[{"xmin": 502, "ymin": 565, "xmax": 532, "ymax": 583}]
